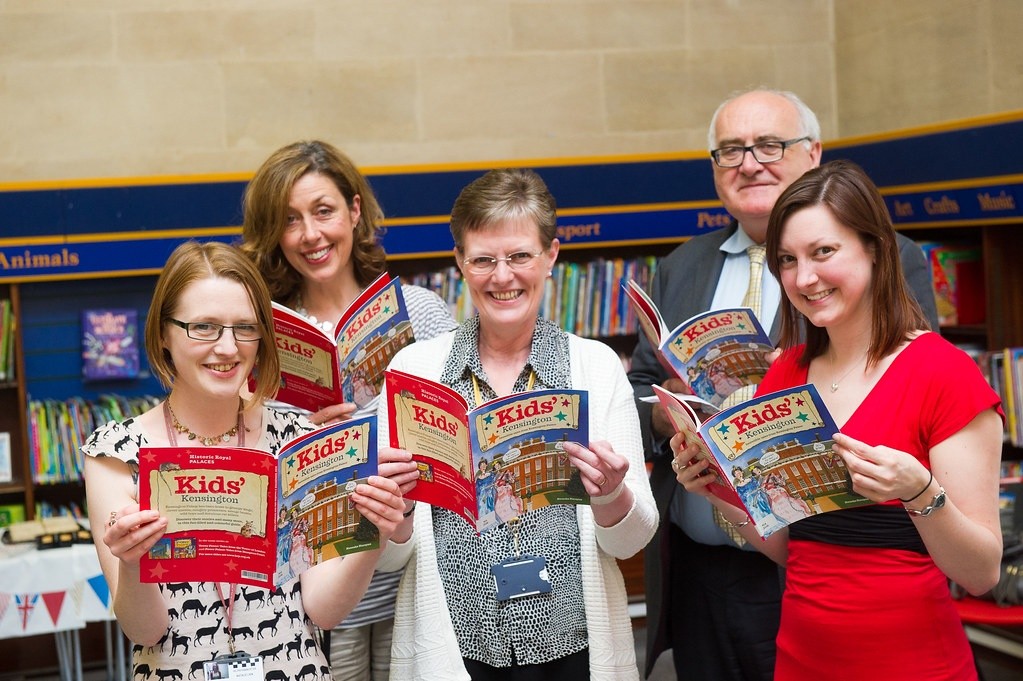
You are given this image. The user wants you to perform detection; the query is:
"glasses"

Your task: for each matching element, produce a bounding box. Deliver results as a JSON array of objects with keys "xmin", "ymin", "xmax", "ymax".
[
  {"xmin": 711, "ymin": 135, "xmax": 812, "ymax": 167},
  {"xmin": 166, "ymin": 315, "xmax": 264, "ymax": 341},
  {"xmin": 456, "ymin": 241, "xmax": 550, "ymax": 274}
]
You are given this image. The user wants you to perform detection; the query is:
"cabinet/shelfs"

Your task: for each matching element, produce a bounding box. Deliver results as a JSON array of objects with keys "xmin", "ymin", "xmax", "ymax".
[{"xmin": 0, "ymin": 221, "xmax": 1023, "ymax": 681}]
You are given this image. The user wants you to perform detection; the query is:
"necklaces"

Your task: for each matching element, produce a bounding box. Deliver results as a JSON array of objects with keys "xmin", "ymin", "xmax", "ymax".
[
  {"xmin": 295, "ymin": 291, "xmax": 335, "ymax": 334},
  {"xmin": 828, "ymin": 342, "xmax": 874, "ymax": 390}
]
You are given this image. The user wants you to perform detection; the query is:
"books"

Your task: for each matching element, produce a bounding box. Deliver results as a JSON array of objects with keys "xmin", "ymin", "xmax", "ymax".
[
  {"xmin": 133, "ymin": 415, "xmax": 383, "ymax": 591},
  {"xmin": 1, "ymin": 243, "xmax": 1023, "ymax": 514},
  {"xmin": 647, "ymin": 379, "xmax": 875, "ymax": 540},
  {"xmin": 376, "ymin": 367, "xmax": 589, "ymax": 536}
]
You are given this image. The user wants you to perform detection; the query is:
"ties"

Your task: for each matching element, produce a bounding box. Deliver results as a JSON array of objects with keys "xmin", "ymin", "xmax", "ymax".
[{"xmin": 708, "ymin": 244, "xmax": 768, "ymax": 548}]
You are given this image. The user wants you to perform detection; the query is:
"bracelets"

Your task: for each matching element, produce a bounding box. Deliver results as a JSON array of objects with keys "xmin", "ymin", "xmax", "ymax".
[
  {"xmin": 898, "ymin": 472, "xmax": 932, "ymax": 503},
  {"xmin": 403, "ymin": 504, "xmax": 416, "ymax": 519},
  {"xmin": 591, "ymin": 479, "xmax": 625, "ymax": 507},
  {"xmin": 721, "ymin": 511, "xmax": 751, "ymax": 530},
  {"xmin": 905, "ymin": 486, "xmax": 946, "ymax": 518}
]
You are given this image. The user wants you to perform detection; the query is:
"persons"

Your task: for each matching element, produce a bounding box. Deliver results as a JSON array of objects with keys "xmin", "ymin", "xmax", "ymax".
[
  {"xmin": 79, "ymin": 141, "xmax": 660, "ymax": 681},
  {"xmin": 668, "ymin": 160, "xmax": 1003, "ymax": 681},
  {"xmin": 625, "ymin": 85, "xmax": 942, "ymax": 681}
]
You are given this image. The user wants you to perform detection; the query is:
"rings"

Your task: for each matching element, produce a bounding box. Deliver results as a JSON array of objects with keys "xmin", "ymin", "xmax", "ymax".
[{"xmin": 671, "ymin": 457, "xmax": 686, "ymax": 470}]
